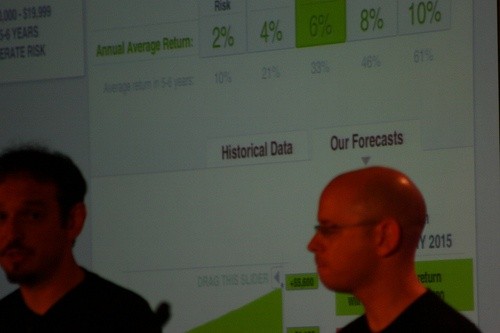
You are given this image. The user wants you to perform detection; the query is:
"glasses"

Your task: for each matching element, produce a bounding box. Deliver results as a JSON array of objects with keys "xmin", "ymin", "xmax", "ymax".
[{"xmin": 314, "ymin": 220, "xmax": 377, "ymax": 236}]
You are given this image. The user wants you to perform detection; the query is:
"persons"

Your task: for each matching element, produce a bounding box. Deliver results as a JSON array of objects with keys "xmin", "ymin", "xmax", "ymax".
[
  {"xmin": 0, "ymin": 145, "xmax": 163, "ymax": 333},
  {"xmin": 307, "ymin": 166, "xmax": 481, "ymax": 333}
]
